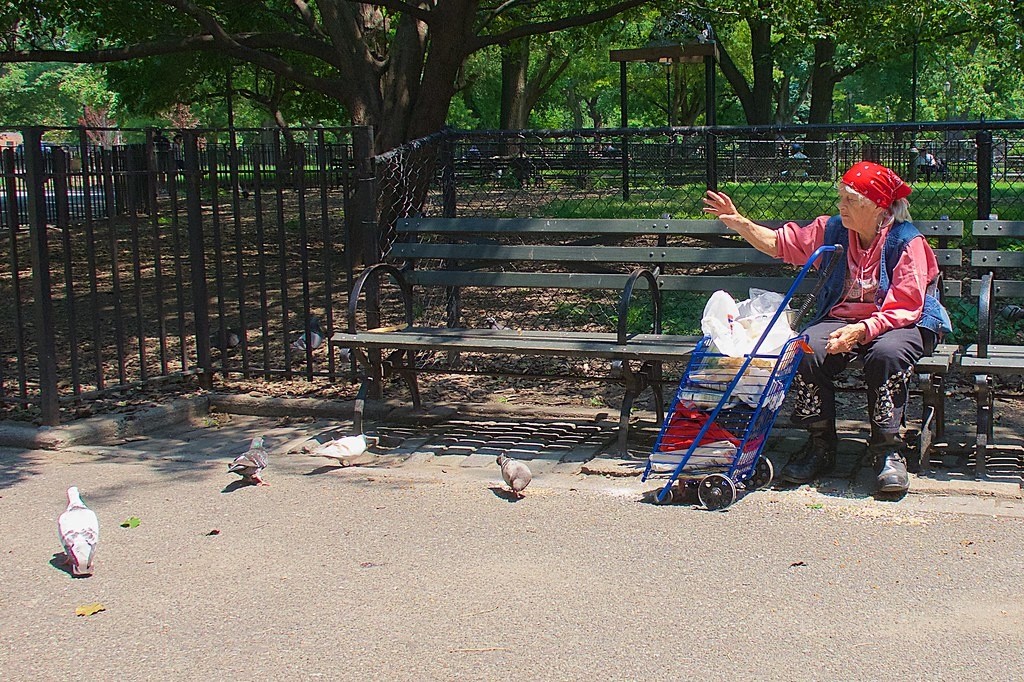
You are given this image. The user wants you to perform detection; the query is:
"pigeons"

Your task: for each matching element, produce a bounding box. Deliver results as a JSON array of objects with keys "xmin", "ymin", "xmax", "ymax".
[
  {"xmin": 207, "ymin": 327, "xmax": 247, "ymax": 359},
  {"xmin": 495, "ymin": 451, "xmax": 532, "ymax": 498},
  {"xmin": 306, "ymin": 433, "xmax": 369, "ymax": 467},
  {"xmin": 226, "ymin": 436, "xmax": 271, "ymax": 487},
  {"xmin": 57, "ymin": 485, "xmax": 100, "ymax": 576},
  {"xmin": 282, "ymin": 314, "xmax": 325, "ymax": 354},
  {"xmin": 483, "ymin": 316, "xmax": 514, "ymax": 332}
]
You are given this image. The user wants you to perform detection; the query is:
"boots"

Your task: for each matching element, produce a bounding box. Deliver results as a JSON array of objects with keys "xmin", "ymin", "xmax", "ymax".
[
  {"xmin": 869, "ymin": 430, "xmax": 910, "ymax": 492},
  {"xmin": 781, "ymin": 416, "xmax": 839, "ymax": 484}
]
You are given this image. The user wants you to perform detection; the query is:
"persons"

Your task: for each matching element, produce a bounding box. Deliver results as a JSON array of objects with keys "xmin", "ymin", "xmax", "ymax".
[
  {"xmin": 152, "ymin": 128, "xmax": 170, "ymax": 193},
  {"xmin": 917, "ymin": 146, "xmax": 936, "ymax": 166},
  {"xmin": 701, "ymin": 162, "xmax": 952, "ymax": 492},
  {"xmin": 467, "ymin": 144, "xmax": 480, "ymax": 159},
  {"xmin": 169, "ymin": 133, "xmax": 184, "ymax": 171},
  {"xmin": 780, "ymin": 144, "xmax": 809, "ymax": 177}
]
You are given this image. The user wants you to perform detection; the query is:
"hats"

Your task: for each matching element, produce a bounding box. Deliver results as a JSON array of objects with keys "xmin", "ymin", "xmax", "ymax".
[{"xmin": 172, "ymin": 133, "xmax": 183, "ymax": 142}]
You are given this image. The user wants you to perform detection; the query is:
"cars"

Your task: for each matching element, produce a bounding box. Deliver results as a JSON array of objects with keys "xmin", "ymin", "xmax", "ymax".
[{"xmin": 16, "ymin": 140, "xmax": 55, "ymax": 152}]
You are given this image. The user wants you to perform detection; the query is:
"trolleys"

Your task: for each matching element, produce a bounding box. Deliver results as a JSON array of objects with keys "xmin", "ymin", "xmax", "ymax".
[{"xmin": 641, "ymin": 244, "xmax": 843, "ymax": 511}]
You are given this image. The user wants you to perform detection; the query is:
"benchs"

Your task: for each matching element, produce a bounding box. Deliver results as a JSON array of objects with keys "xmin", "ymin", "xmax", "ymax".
[
  {"xmin": 961, "ymin": 213, "xmax": 1024, "ymax": 478},
  {"xmin": 330, "ymin": 215, "xmax": 964, "ymax": 471}
]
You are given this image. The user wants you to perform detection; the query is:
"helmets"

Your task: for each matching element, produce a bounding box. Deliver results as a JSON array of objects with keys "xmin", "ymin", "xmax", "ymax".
[{"xmin": 790, "ymin": 143, "xmax": 801, "ymax": 152}]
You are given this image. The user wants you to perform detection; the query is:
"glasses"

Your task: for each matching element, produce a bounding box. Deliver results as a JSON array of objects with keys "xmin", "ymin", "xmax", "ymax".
[{"xmin": 178, "ymin": 138, "xmax": 183, "ymax": 141}]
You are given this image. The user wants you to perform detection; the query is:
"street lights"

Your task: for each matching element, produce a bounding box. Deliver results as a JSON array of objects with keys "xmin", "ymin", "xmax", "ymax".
[
  {"xmin": 885, "ymin": 106, "xmax": 891, "ymax": 170},
  {"xmin": 846, "ymin": 90, "xmax": 853, "ymax": 170},
  {"xmin": 831, "ymin": 104, "xmax": 836, "ymax": 180},
  {"xmin": 662, "ymin": 63, "xmax": 673, "ymax": 183},
  {"xmin": 943, "ymin": 82, "xmax": 951, "ymax": 180}
]
